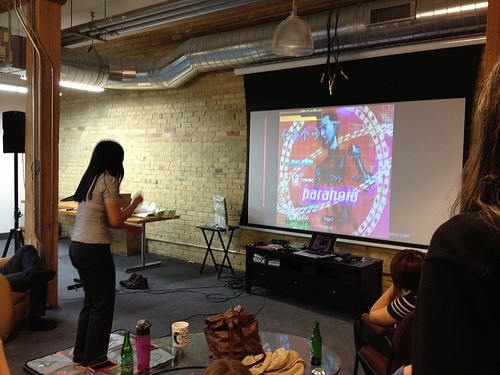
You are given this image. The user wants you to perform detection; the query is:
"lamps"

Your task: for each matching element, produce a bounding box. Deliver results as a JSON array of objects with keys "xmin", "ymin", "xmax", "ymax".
[{"xmin": 271, "ymin": 0, "xmax": 314, "ymax": 57}]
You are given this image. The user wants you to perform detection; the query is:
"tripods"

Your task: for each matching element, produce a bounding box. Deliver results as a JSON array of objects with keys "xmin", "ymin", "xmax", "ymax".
[{"xmin": 2, "ymin": 153, "xmax": 24, "ymax": 258}]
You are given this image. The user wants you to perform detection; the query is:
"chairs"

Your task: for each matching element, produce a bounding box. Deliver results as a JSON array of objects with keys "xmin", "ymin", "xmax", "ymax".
[{"xmin": 353, "ymin": 311, "xmax": 414, "ymax": 375}]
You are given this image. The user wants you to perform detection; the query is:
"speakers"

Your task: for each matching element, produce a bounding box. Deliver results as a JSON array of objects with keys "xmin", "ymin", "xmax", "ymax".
[{"xmin": 2, "ymin": 111, "xmax": 26, "ymax": 153}]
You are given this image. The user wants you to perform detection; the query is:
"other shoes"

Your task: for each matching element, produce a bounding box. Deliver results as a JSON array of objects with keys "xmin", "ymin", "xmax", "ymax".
[
  {"xmin": 127, "ymin": 275, "xmax": 148, "ymax": 289},
  {"xmin": 119, "ymin": 273, "xmax": 139, "ymax": 286},
  {"xmin": 28, "ymin": 270, "xmax": 56, "ymax": 285},
  {"xmin": 30, "ymin": 319, "xmax": 56, "ymax": 331}
]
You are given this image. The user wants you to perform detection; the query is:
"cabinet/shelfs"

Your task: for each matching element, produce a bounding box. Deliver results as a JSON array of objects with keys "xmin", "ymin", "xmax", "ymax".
[{"xmin": 245, "ymin": 244, "xmax": 383, "ymax": 318}]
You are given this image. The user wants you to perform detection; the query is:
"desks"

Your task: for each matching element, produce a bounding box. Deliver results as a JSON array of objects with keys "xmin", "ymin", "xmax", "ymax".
[
  {"xmin": 58, "ymin": 209, "xmax": 180, "ymax": 273},
  {"xmin": 84, "ymin": 333, "xmax": 342, "ymax": 375},
  {"xmin": 196, "ymin": 226, "xmax": 239, "ymax": 278}
]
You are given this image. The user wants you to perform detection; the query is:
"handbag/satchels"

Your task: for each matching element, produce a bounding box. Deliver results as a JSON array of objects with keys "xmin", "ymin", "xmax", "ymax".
[{"xmin": 205, "ymin": 304, "xmax": 266, "ymax": 370}]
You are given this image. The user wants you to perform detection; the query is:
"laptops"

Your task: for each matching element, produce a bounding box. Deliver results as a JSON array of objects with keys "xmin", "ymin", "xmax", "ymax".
[{"xmin": 293, "ymin": 232, "xmax": 338, "ymax": 259}]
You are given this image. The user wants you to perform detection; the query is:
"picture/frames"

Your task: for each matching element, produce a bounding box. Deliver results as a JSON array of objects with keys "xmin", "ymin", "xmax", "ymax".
[{"xmin": 309, "ymin": 232, "xmax": 337, "ymax": 254}]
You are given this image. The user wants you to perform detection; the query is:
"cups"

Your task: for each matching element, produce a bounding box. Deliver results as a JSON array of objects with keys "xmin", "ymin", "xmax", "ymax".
[{"xmin": 171, "ymin": 321, "xmax": 189, "ymax": 347}]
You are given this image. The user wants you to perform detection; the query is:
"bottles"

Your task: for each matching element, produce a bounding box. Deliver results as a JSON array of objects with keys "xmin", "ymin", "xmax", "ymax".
[
  {"xmin": 120, "ymin": 332, "xmax": 133, "ymax": 375},
  {"xmin": 311, "ymin": 320, "xmax": 322, "ymax": 366},
  {"xmin": 136, "ymin": 320, "xmax": 152, "ymax": 372}
]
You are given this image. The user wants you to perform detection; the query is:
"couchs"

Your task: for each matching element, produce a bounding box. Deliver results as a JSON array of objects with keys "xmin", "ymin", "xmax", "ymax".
[{"xmin": 0, "ymin": 249, "xmax": 39, "ymax": 340}]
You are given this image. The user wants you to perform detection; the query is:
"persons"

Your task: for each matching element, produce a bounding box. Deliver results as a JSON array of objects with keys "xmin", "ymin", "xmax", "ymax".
[
  {"xmin": 201, "ymin": 358, "xmax": 254, "ymax": 375},
  {"xmin": 353, "ymin": 249, "xmax": 425, "ymax": 375},
  {"xmin": 69, "ymin": 141, "xmax": 144, "ymax": 368},
  {"xmin": 0, "ymin": 245, "xmax": 58, "ymax": 332},
  {"xmin": 411, "ymin": 60, "xmax": 500, "ymax": 375}
]
